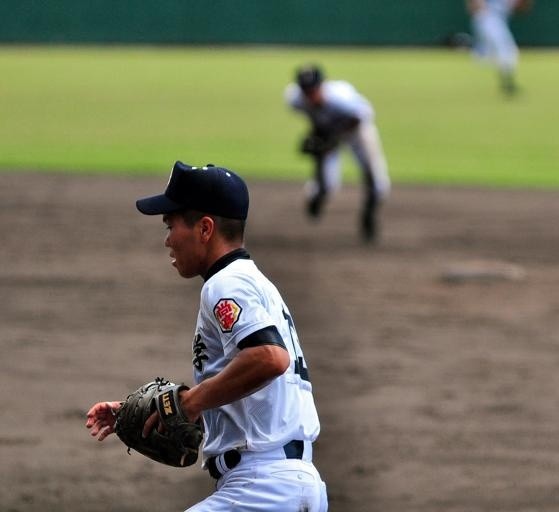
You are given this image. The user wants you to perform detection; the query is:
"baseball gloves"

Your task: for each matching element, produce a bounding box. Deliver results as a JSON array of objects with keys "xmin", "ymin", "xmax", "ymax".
[
  {"xmin": 115, "ymin": 376, "xmax": 205, "ymax": 467},
  {"xmin": 303, "ymin": 124, "xmax": 340, "ymax": 155}
]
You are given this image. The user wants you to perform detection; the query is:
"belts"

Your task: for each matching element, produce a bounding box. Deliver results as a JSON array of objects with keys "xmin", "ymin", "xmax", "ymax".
[{"xmin": 206, "ymin": 440, "xmax": 303, "ymax": 479}]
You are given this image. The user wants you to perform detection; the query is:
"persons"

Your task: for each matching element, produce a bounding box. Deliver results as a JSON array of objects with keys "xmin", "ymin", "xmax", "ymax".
[
  {"xmin": 467, "ymin": 0, "xmax": 533, "ymax": 96},
  {"xmin": 286, "ymin": 62, "xmax": 390, "ymax": 244},
  {"xmin": 86, "ymin": 160, "xmax": 329, "ymax": 511}
]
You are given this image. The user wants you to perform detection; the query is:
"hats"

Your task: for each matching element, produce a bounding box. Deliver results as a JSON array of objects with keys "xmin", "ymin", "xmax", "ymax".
[{"xmin": 136, "ymin": 161, "xmax": 248, "ymax": 221}]
